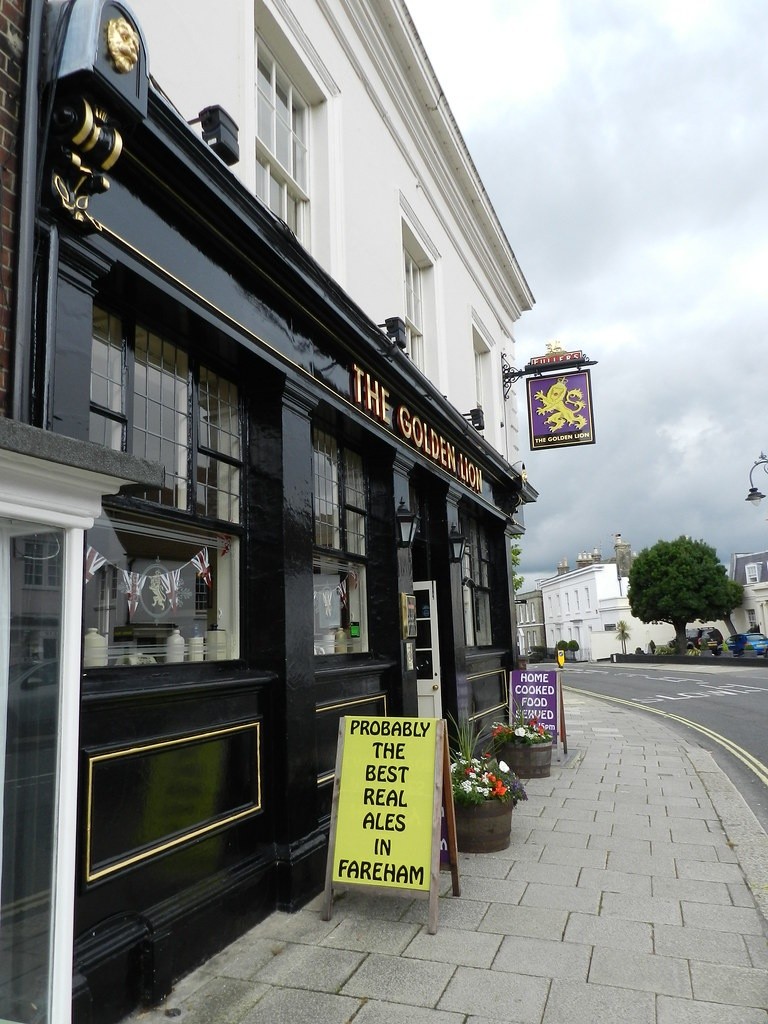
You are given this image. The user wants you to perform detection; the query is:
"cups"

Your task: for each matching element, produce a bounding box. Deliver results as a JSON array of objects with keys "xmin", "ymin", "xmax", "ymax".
[
  {"xmin": 323, "ymin": 635, "xmax": 335, "ymax": 653},
  {"xmin": 188, "ymin": 638, "xmax": 204, "ymax": 660}
]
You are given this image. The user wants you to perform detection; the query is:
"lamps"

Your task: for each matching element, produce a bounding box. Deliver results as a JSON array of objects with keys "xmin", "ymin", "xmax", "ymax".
[
  {"xmin": 449, "ymin": 521, "xmax": 468, "ymax": 563},
  {"xmin": 380, "ymin": 318, "xmax": 411, "ymax": 351},
  {"xmin": 463, "ymin": 407, "xmax": 485, "ymax": 430},
  {"xmin": 187, "ymin": 106, "xmax": 239, "ymax": 165},
  {"xmin": 397, "ymin": 497, "xmax": 422, "ymax": 549}
]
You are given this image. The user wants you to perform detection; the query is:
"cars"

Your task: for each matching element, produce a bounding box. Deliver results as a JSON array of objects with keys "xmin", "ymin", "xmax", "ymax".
[{"xmin": 718, "ymin": 634, "xmax": 768, "ymax": 658}]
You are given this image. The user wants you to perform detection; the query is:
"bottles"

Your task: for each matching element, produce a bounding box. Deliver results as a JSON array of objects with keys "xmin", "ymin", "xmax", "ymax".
[
  {"xmin": 350, "ymin": 622, "xmax": 360, "ymax": 637},
  {"xmin": 84, "ymin": 628, "xmax": 109, "ymax": 668},
  {"xmin": 337, "ymin": 628, "xmax": 347, "ymax": 653},
  {"xmin": 167, "ymin": 629, "xmax": 185, "ymax": 662},
  {"xmin": 204, "ymin": 624, "xmax": 226, "ymax": 660}
]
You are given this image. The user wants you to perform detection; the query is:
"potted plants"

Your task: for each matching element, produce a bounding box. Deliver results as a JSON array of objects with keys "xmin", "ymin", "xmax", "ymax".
[
  {"xmin": 700, "ymin": 641, "xmax": 712, "ymax": 658},
  {"xmin": 721, "ymin": 638, "xmax": 733, "ymax": 657},
  {"xmin": 743, "ymin": 644, "xmax": 757, "ymax": 657}
]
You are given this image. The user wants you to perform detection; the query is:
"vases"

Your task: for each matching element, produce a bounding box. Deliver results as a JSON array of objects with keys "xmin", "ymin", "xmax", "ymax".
[
  {"xmin": 454, "ymin": 797, "xmax": 513, "ymax": 854},
  {"xmin": 508, "ymin": 741, "xmax": 552, "ymax": 779}
]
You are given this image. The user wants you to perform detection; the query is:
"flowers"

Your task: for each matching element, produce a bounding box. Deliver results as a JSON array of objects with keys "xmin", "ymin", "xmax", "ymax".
[
  {"xmin": 438, "ymin": 706, "xmax": 529, "ymax": 807},
  {"xmin": 488, "ymin": 699, "xmax": 554, "ymax": 748}
]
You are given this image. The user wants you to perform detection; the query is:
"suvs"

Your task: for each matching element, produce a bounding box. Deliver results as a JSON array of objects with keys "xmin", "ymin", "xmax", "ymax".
[{"xmin": 668, "ymin": 627, "xmax": 723, "ymax": 655}]
